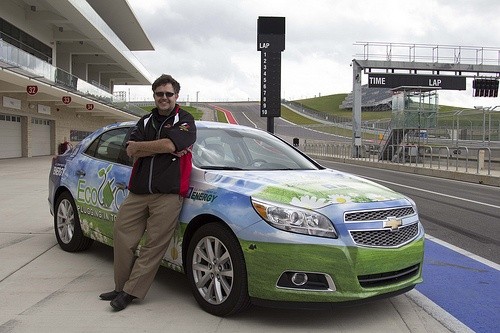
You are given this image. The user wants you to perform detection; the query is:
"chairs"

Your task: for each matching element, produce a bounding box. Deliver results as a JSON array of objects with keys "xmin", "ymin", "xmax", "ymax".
[
  {"xmin": 193, "ymin": 142, "xmax": 235, "ymax": 170},
  {"xmin": 101, "ymin": 141, "xmax": 124, "ymax": 164}
]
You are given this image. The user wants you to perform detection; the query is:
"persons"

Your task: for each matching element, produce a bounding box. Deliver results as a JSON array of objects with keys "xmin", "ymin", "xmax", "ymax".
[{"xmin": 99, "ymin": 73, "xmax": 198, "ymax": 311}]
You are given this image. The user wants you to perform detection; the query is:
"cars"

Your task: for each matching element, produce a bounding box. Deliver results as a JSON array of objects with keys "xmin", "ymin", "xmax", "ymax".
[{"xmin": 45, "ymin": 119, "xmax": 426, "ymax": 318}]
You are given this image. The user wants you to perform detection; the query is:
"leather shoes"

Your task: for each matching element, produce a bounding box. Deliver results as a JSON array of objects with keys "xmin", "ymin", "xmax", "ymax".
[
  {"xmin": 99, "ymin": 289, "xmax": 122, "ymax": 300},
  {"xmin": 110, "ymin": 291, "xmax": 133, "ymax": 310}
]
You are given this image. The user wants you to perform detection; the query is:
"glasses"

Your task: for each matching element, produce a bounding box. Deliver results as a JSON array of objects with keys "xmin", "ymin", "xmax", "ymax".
[{"xmin": 154, "ymin": 91, "xmax": 175, "ymax": 97}]
